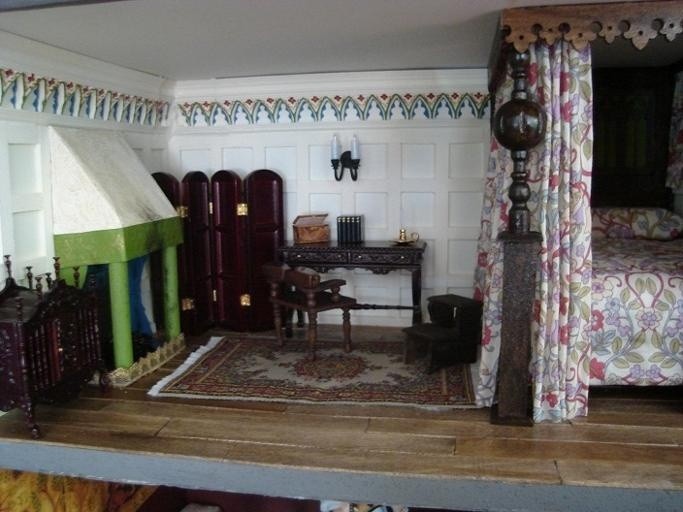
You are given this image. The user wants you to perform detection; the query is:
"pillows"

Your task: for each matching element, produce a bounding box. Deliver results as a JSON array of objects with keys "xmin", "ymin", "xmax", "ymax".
[{"xmin": 590, "ymin": 205, "xmax": 680, "ymax": 241}]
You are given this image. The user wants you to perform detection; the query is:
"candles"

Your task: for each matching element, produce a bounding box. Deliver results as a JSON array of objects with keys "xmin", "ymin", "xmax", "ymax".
[
  {"xmin": 330, "ymin": 134, "xmax": 339, "ymax": 159},
  {"xmin": 350, "ymin": 134, "xmax": 360, "ymax": 159}
]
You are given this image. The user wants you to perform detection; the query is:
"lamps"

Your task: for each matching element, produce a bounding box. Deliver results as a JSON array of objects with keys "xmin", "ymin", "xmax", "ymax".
[{"xmin": 329, "ymin": 133, "xmax": 361, "ymax": 183}]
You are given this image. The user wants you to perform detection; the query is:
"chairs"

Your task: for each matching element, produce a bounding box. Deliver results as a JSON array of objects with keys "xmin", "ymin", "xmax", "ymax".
[{"xmin": 271, "ymin": 269, "xmax": 356, "ymax": 360}]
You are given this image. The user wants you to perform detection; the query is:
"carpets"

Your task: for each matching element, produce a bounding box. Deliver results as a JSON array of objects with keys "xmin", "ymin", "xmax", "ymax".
[{"xmin": 143, "ymin": 335, "xmax": 478, "ymax": 410}]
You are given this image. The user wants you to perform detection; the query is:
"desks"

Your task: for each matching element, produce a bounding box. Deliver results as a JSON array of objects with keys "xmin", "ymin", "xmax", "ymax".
[{"xmin": 278, "ymin": 238, "xmax": 428, "ymax": 337}]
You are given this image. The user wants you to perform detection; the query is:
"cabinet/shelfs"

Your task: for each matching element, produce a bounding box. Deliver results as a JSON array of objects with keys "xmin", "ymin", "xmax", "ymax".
[{"xmin": 0, "ymin": 250, "xmax": 112, "ymax": 440}]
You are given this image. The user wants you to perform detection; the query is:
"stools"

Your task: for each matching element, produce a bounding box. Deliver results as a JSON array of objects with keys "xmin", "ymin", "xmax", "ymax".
[
  {"xmin": 424, "ymin": 292, "xmax": 482, "ymax": 364},
  {"xmin": 401, "ymin": 321, "xmax": 456, "ymax": 371}
]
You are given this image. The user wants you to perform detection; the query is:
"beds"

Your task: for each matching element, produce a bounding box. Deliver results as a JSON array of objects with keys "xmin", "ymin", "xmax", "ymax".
[{"xmin": 473, "ymin": 0, "xmax": 682, "ymax": 430}]
